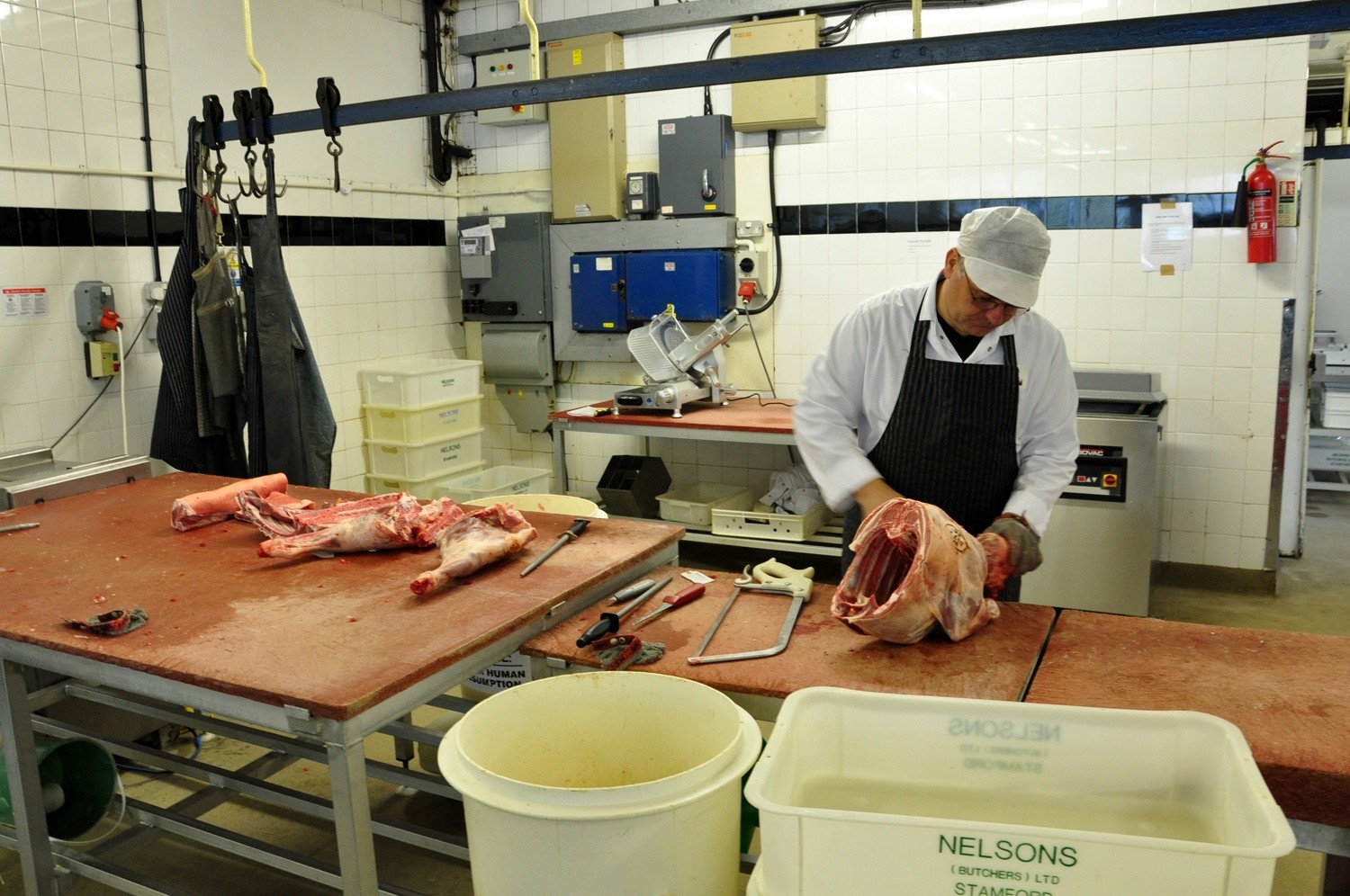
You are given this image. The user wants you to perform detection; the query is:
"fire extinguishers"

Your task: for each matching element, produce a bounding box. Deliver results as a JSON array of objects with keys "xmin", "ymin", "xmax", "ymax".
[{"xmin": 1248, "ymin": 141, "xmax": 1291, "ymax": 264}]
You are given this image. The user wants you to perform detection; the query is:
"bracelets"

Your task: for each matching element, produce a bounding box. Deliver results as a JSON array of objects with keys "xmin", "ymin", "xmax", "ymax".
[{"xmin": 994, "ymin": 512, "xmax": 1043, "ymax": 544}]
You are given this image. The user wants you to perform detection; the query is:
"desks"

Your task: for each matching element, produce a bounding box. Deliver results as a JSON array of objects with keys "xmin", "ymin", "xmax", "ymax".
[
  {"xmin": 544, "ymin": 385, "xmax": 865, "ymax": 580},
  {"xmin": 517, "ymin": 560, "xmax": 1058, "ymax": 879},
  {"xmin": 1025, "ymin": 604, "xmax": 1349, "ymax": 862},
  {"xmin": 0, "ymin": 468, "xmax": 691, "ymax": 896}
]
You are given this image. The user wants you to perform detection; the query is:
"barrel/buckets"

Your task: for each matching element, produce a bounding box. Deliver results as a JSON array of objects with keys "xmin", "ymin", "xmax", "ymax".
[{"xmin": 432, "ymin": 666, "xmax": 766, "ymax": 896}]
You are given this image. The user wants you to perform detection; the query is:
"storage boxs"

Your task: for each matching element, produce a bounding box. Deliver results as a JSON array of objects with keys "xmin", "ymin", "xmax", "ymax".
[
  {"xmin": 709, "ymin": 488, "xmax": 832, "ymax": 543},
  {"xmin": 652, "ymin": 478, "xmax": 751, "ymax": 524},
  {"xmin": 739, "ymin": 682, "xmax": 1299, "ymax": 893},
  {"xmin": 356, "ymin": 355, "xmax": 555, "ymax": 508},
  {"xmin": 595, "ymin": 454, "xmax": 673, "ymax": 519},
  {"xmin": 1307, "ymin": 346, "xmax": 1350, "ymax": 473}
]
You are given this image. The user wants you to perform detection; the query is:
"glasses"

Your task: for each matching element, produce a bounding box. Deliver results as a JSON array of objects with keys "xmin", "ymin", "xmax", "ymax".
[{"xmin": 963, "ymin": 271, "xmax": 1032, "ymax": 316}]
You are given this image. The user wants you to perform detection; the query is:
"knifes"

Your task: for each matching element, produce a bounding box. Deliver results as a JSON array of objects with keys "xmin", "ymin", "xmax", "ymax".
[{"xmin": 633, "ymin": 582, "xmax": 706, "ymax": 629}]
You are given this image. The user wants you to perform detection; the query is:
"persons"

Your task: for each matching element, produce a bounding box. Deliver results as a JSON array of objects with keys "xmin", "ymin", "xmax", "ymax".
[{"xmin": 793, "ymin": 206, "xmax": 1081, "ymax": 603}]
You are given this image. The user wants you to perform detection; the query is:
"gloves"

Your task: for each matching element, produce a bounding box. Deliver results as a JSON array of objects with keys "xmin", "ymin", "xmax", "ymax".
[{"xmin": 985, "ymin": 518, "xmax": 1043, "ymax": 574}]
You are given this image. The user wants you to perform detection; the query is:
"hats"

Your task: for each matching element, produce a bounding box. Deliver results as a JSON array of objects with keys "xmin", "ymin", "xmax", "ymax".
[{"xmin": 953, "ymin": 206, "xmax": 1050, "ymax": 306}]
